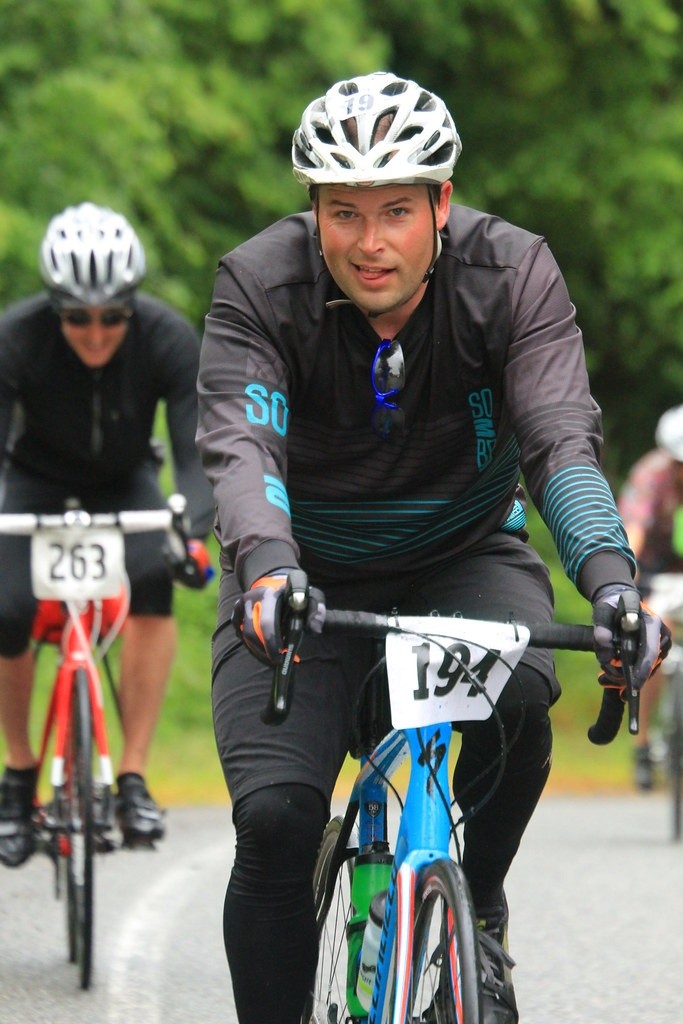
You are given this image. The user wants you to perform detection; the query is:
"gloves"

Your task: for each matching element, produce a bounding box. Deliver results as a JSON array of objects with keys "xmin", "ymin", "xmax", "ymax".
[
  {"xmin": 175, "ymin": 539, "xmax": 211, "ymax": 590},
  {"xmin": 592, "ymin": 584, "xmax": 673, "ymax": 704},
  {"xmin": 232, "ymin": 568, "xmax": 325, "ymax": 666}
]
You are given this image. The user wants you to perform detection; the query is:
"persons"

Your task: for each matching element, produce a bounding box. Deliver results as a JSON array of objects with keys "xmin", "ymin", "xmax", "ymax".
[
  {"xmin": 195, "ymin": 72, "xmax": 672, "ymax": 1024},
  {"xmin": 0, "ymin": 201, "xmax": 216, "ymax": 866},
  {"xmin": 617, "ymin": 405, "xmax": 683, "ymax": 790}
]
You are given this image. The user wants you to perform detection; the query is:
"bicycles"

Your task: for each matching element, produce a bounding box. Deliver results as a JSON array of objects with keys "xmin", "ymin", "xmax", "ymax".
[
  {"xmin": 0, "ymin": 494, "xmax": 187, "ymax": 989},
  {"xmin": 638, "ymin": 576, "xmax": 683, "ymax": 840},
  {"xmin": 262, "ymin": 570, "xmax": 644, "ymax": 1024}
]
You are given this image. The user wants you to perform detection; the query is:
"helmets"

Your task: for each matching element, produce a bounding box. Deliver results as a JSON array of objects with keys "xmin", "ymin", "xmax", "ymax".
[
  {"xmin": 655, "ymin": 403, "xmax": 683, "ymax": 461},
  {"xmin": 39, "ymin": 202, "xmax": 146, "ymax": 306},
  {"xmin": 291, "ymin": 70, "xmax": 460, "ymax": 184}
]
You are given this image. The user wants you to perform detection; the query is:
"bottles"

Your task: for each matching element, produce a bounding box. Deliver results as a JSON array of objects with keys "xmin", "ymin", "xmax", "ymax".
[
  {"xmin": 355, "ymin": 888, "xmax": 388, "ymax": 1013},
  {"xmin": 345, "ymin": 840, "xmax": 395, "ymax": 1019}
]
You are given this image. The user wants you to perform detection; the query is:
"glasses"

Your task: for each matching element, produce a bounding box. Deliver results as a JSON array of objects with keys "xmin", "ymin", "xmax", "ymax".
[
  {"xmin": 53, "ymin": 305, "xmax": 134, "ymax": 326},
  {"xmin": 372, "ymin": 338, "xmax": 407, "ymax": 440}
]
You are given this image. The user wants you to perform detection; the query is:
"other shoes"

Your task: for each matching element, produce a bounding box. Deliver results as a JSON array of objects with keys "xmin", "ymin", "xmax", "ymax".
[{"xmin": 636, "ymin": 748, "xmax": 653, "ymax": 788}]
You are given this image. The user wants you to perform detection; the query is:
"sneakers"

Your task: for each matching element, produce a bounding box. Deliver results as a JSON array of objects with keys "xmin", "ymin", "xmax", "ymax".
[
  {"xmin": 0, "ymin": 770, "xmax": 40, "ymax": 866},
  {"xmin": 119, "ymin": 775, "xmax": 165, "ymax": 840},
  {"xmin": 428, "ymin": 884, "xmax": 519, "ymax": 1024}
]
